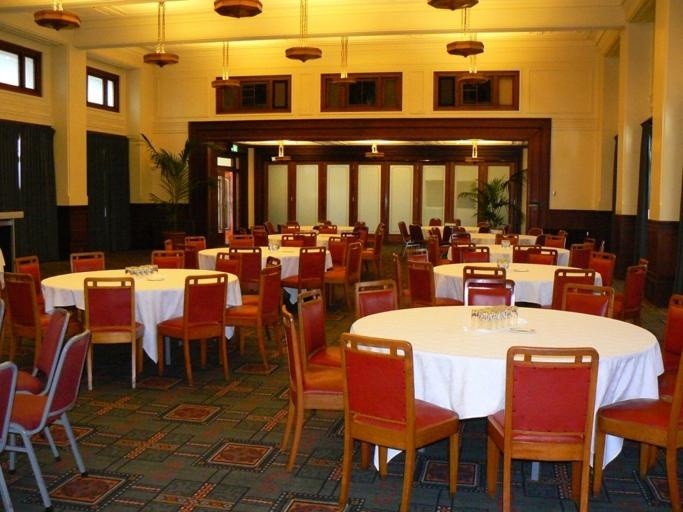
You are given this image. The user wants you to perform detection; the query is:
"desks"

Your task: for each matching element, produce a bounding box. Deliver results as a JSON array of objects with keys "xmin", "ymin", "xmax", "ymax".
[
  {"xmin": 41, "ymin": 268, "xmax": 238, "ymax": 374},
  {"xmin": 349, "ymin": 306, "xmax": 656, "ymax": 496}
]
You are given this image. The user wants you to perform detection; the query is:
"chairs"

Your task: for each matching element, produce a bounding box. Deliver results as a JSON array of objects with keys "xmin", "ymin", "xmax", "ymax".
[
  {"xmin": 462, "ymin": 266, "xmax": 516, "ymax": 307},
  {"xmin": 297, "ymin": 288, "xmax": 343, "ymax": 367},
  {"xmin": 0, "ymin": 308, "xmax": 92, "ymax": 511},
  {"xmin": 651, "ymin": 295, "xmax": 683, "ymax": 466},
  {"xmin": 486, "ymin": 346, "xmax": 600, "ymax": 510},
  {"xmin": 594, "ymin": 358, "xmax": 683, "ymax": 512},
  {"xmin": 278, "ymin": 304, "xmax": 371, "ymax": 473},
  {"xmin": 569, "ymin": 239, "xmax": 649, "ymax": 327},
  {"xmin": 336, "ymin": 333, "xmax": 458, "ymax": 512},
  {"xmin": 156, "ymin": 273, "xmax": 230, "ymax": 387},
  {"xmin": 83, "ymin": 278, "xmax": 144, "ymax": 390},
  {"xmin": 355, "ymin": 280, "xmax": 399, "ymax": 314},
  {"xmin": 0, "ymin": 257, "xmax": 79, "ymax": 372},
  {"xmin": 69, "ymin": 217, "xmax": 593, "ymax": 305},
  {"xmin": 542, "ymin": 269, "xmax": 614, "ymax": 319},
  {"xmin": 224, "ymin": 264, "xmax": 280, "ymax": 369}
]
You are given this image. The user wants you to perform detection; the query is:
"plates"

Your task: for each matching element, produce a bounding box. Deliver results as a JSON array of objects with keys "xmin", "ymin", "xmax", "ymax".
[
  {"xmin": 511, "ymin": 327, "xmax": 534, "ymax": 334},
  {"xmin": 147, "ymin": 275, "xmax": 164, "ymax": 281},
  {"xmin": 514, "ymin": 269, "xmax": 530, "ymax": 272}
]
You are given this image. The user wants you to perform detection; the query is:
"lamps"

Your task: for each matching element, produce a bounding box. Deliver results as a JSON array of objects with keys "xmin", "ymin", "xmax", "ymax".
[
  {"xmin": 325, "ymin": 35, "xmax": 358, "ymax": 89},
  {"xmin": 211, "ymin": 0, "xmax": 265, "ymax": 19},
  {"xmin": 31, "ymin": 0, "xmax": 85, "ymax": 29},
  {"xmin": 209, "ymin": 39, "xmax": 244, "ymax": 92},
  {"xmin": 363, "ymin": 143, "xmax": 385, "ymax": 161},
  {"xmin": 141, "ymin": 0, "xmax": 180, "ymax": 68},
  {"xmin": 462, "ymin": 144, "xmax": 486, "ymax": 165},
  {"xmin": 457, "ymin": 32, "xmax": 490, "ymax": 86},
  {"xmin": 268, "ymin": 143, "xmax": 292, "ymax": 165},
  {"xmin": 423, "ymin": 0, "xmax": 483, "ymax": 12},
  {"xmin": 282, "ymin": 0, "xmax": 324, "ymax": 62},
  {"xmin": 446, "ymin": 7, "xmax": 485, "ymax": 59}
]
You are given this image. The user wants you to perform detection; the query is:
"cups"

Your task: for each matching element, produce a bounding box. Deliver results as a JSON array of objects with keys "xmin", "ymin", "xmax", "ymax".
[
  {"xmin": 496, "ymin": 239, "xmax": 511, "ymax": 271},
  {"xmin": 268, "ymin": 239, "xmax": 279, "ymax": 253},
  {"xmin": 123, "ymin": 264, "xmax": 160, "ymax": 281},
  {"xmin": 467, "ymin": 303, "xmax": 519, "ymax": 332}
]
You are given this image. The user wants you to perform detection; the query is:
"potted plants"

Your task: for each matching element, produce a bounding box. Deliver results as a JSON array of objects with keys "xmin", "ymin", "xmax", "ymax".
[{"xmin": 137, "ymin": 130, "xmax": 224, "ymax": 249}]
